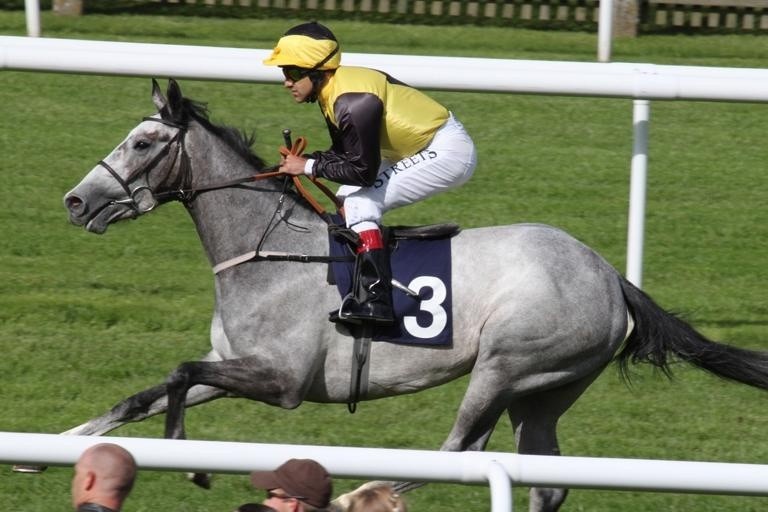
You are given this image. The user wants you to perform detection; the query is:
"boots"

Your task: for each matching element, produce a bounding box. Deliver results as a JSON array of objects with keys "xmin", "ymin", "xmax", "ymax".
[{"xmin": 328, "ymin": 248, "xmax": 395, "ymax": 324}]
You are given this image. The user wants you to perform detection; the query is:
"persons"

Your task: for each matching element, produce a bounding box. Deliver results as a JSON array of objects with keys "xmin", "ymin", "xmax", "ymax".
[
  {"xmin": 236, "ymin": 504, "xmax": 276, "ymax": 512},
  {"xmin": 263, "ymin": 22, "xmax": 476, "ymax": 322},
  {"xmin": 72, "ymin": 443, "xmax": 137, "ymax": 512}
]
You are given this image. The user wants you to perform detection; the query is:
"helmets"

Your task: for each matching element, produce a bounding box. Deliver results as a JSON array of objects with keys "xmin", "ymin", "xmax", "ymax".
[{"xmin": 261, "ymin": 20, "xmax": 341, "ymax": 71}]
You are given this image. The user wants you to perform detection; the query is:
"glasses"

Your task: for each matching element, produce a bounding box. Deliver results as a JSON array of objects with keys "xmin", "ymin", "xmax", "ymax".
[
  {"xmin": 265, "ymin": 490, "xmax": 305, "ymax": 500},
  {"xmin": 282, "ymin": 67, "xmax": 303, "ymax": 82}
]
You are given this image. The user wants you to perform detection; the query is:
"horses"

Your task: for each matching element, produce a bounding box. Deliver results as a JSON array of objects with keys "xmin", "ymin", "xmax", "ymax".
[{"xmin": 6, "ymin": 70, "xmax": 768, "ymax": 512}]
